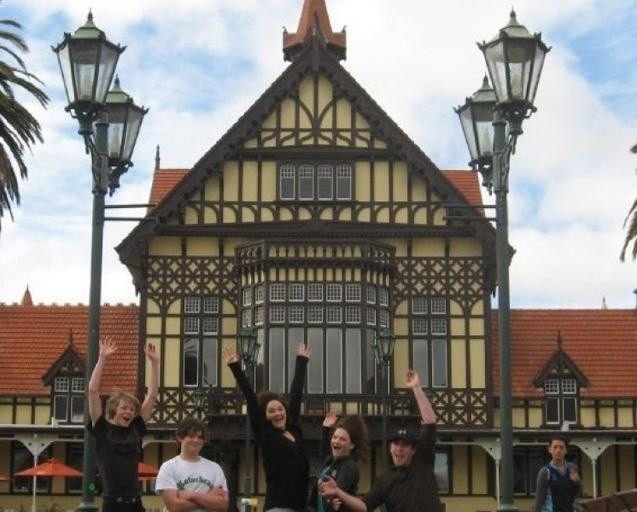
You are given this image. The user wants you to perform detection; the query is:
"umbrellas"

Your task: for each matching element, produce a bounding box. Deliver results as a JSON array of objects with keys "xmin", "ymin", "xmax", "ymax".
[
  {"xmin": 95, "ymin": 461, "xmax": 160, "ymax": 482},
  {"xmin": 15, "ymin": 456, "xmax": 84, "ymax": 500}
]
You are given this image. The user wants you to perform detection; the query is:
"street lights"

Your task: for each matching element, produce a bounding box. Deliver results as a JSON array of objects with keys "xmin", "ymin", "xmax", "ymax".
[
  {"xmin": 49, "ymin": 7, "xmax": 151, "ymax": 512},
  {"xmin": 451, "ymin": 5, "xmax": 554, "ymax": 512}
]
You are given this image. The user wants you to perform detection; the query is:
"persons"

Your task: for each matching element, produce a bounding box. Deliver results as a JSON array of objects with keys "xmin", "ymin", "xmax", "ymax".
[
  {"xmin": 154, "ymin": 416, "xmax": 230, "ymax": 511},
  {"xmin": 532, "ymin": 434, "xmax": 581, "ymax": 512},
  {"xmin": 308, "ymin": 407, "xmax": 368, "ymax": 511},
  {"xmin": 220, "ymin": 343, "xmax": 312, "ymax": 512},
  {"xmin": 318, "ymin": 369, "xmax": 445, "ymax": 511},
  {"xmin": 83, "ymin": 333, "xmax": 158, "ymax": 511}
]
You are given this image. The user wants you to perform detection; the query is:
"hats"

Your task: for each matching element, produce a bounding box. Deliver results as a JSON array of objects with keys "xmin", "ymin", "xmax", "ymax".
[{"xmin": 389, "ymin": 428, "xmax": 416, "ymax": 446}]
